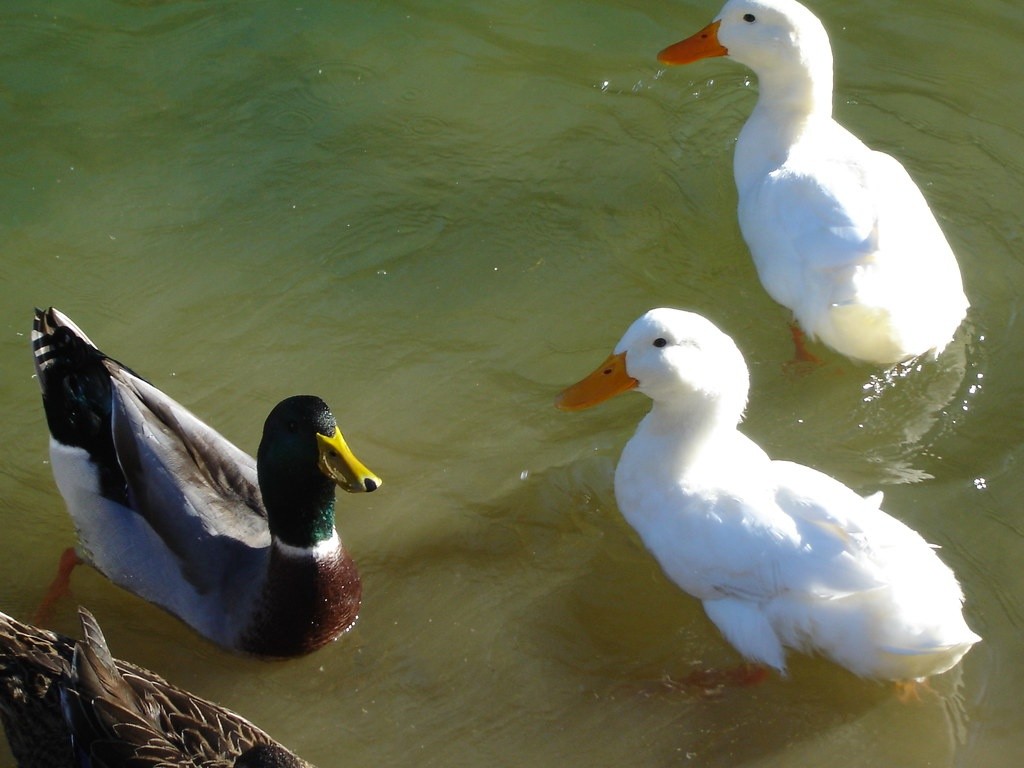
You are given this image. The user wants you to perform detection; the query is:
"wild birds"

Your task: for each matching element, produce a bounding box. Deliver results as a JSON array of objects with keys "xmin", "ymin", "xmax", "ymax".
[
  {"xmin": 0, "ymin": 612, "xmax": 318, "ymax": 768},
  {"xmin": 32, "ymin": 306, "xmax": 383, "ymax": 662}
]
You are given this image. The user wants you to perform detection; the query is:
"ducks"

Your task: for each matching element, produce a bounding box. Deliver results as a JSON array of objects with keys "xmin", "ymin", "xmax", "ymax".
[
  {"xmin": 657, "ymin": 0, "xmax": 971, "ymax": 365},
  {"xmin": 552, "ymin": 307, "xmax": 982, "ymax": 700}
]
